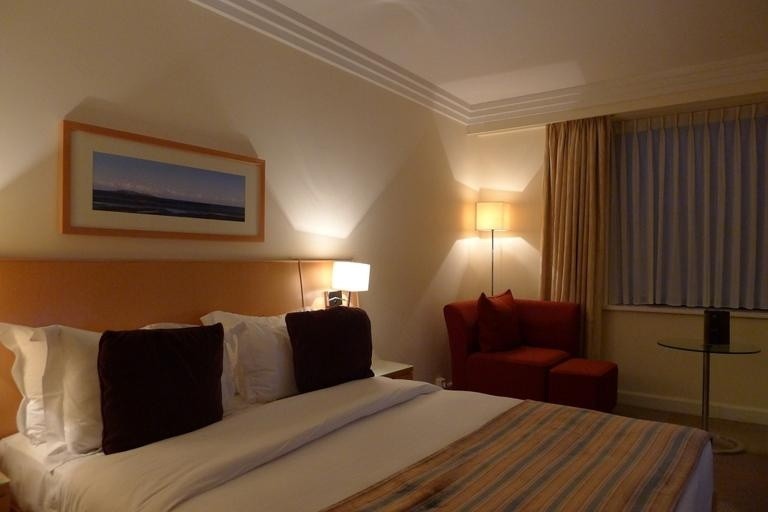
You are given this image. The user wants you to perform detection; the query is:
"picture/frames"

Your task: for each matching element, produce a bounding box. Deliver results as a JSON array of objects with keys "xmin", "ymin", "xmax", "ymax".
[{"xmin": 58, "ymin": 120, "xmax": 265, "ymax": 242}]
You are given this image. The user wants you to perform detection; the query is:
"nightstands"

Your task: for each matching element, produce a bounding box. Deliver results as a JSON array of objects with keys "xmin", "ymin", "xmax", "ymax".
[
  {"xmin": 0, "ymin": 472, "xmax": 10, "ymax": 512},
  {"xmin": 371, "ymin": 360, "xmax": 414, "ymax": 380}
]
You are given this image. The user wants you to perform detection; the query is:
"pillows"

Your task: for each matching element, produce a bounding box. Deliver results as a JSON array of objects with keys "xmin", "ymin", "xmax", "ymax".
[{"xmin": 478, "ymin": 290, "xmax": 519, "ymax": 352}]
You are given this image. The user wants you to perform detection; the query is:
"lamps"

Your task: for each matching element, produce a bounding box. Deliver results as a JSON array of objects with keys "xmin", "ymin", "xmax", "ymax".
[
  {"xmin": 476, "ymin": 201, "xmax": 508, "ymax": 297},
  {"xmin": 333, "ymin": 261, "xmax": 370, "ymax": 307}
]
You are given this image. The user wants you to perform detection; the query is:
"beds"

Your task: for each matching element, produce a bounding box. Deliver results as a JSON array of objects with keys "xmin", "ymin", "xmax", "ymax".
[{"xmin": 0, "ymin": 259, "xmax": 716, "ymax": 512}]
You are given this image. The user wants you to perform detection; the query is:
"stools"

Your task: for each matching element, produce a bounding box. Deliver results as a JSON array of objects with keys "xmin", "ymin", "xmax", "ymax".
[{"xmin": 548, "ymin": 358, "xmax": 617, "ymax": 412}]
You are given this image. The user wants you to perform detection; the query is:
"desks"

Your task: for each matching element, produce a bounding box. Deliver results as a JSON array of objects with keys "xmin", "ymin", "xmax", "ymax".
[{"xmin": 657, "ymin": 337, "xmax": 761, "ymax": 454}]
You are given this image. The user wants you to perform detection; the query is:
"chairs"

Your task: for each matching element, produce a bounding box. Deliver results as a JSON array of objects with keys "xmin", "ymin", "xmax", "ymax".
[{"xmin": 443, "ymin": 299, "xmax": 581, "ymax": 401}]
